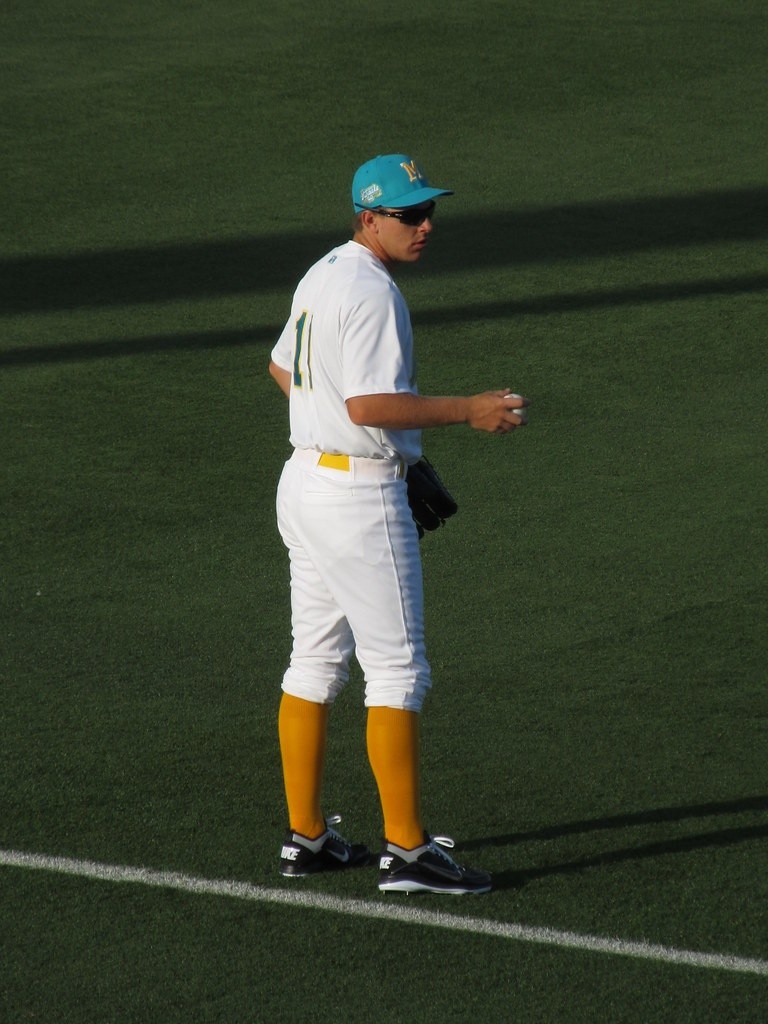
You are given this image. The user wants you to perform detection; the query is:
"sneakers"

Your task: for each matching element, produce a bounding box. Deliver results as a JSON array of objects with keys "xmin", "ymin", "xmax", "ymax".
[
  {"xmin": 377, "ymin": 831, "xmax": 503, "ymax": 896},
  {"xmin": 277, "ymin": 815, "xmax": 372, "ymax": 876}
]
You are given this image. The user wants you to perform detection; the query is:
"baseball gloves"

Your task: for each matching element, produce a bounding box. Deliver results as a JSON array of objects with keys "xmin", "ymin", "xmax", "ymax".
[{"xmin": 405, "ymin": 456, "xmax": 459, "ymax": 539}]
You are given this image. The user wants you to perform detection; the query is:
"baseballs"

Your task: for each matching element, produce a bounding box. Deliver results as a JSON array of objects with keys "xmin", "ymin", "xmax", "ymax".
[{"xmin": 504, "ymin": 392, "xmax": 527, "ymax": 420}]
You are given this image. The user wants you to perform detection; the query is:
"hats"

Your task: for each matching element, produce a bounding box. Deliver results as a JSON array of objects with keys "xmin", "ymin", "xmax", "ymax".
[{"xmin": 352, "ymin": 154, "xmax": 456, "ymax": 212}]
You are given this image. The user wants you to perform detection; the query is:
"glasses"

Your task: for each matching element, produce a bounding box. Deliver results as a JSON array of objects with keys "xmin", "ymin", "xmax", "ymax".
[{"xmin": 354, "ymin": 198, "xmax": 438, "ymax": 226}]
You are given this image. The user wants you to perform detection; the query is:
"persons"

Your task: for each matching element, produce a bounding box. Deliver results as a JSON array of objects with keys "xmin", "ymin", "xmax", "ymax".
[{"xmin": 269, "ymin": 155, "xmax": 530, "ymax": 897}]
